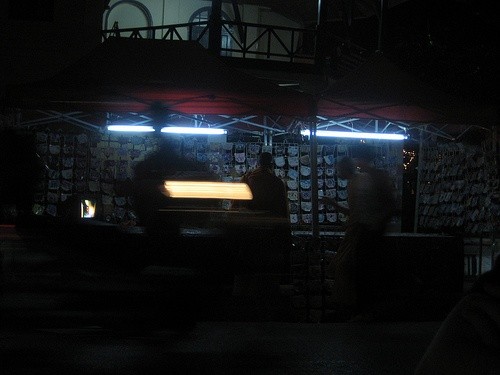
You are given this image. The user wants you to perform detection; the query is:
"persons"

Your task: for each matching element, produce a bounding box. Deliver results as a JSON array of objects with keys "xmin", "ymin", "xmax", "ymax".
[
  {"xmin": 338, "ymin": 145, "xmax": 400, "ymax": 268},
  {"xmin": 226, "ymin": 152, "xmax": 292, "ymax": 257}
]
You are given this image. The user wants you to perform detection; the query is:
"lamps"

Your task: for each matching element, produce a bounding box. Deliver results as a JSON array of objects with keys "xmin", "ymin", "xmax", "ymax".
[
  {"xmin": 301, "ymin": 128, "xmax": 410, "ymax": 142},
  {"xmin": 161, "ymin": 126, "xmax": 228, "ymax": 135},
  {"xmin": 106, "ymin": 124, "xmax": 155, "ymax": 132}
]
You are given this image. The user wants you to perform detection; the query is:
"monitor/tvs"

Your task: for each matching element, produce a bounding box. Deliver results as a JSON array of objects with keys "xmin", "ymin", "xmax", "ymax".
[{"xmin": 79, "ymin": 200, "xmax": 98, "ymax": 218}]
